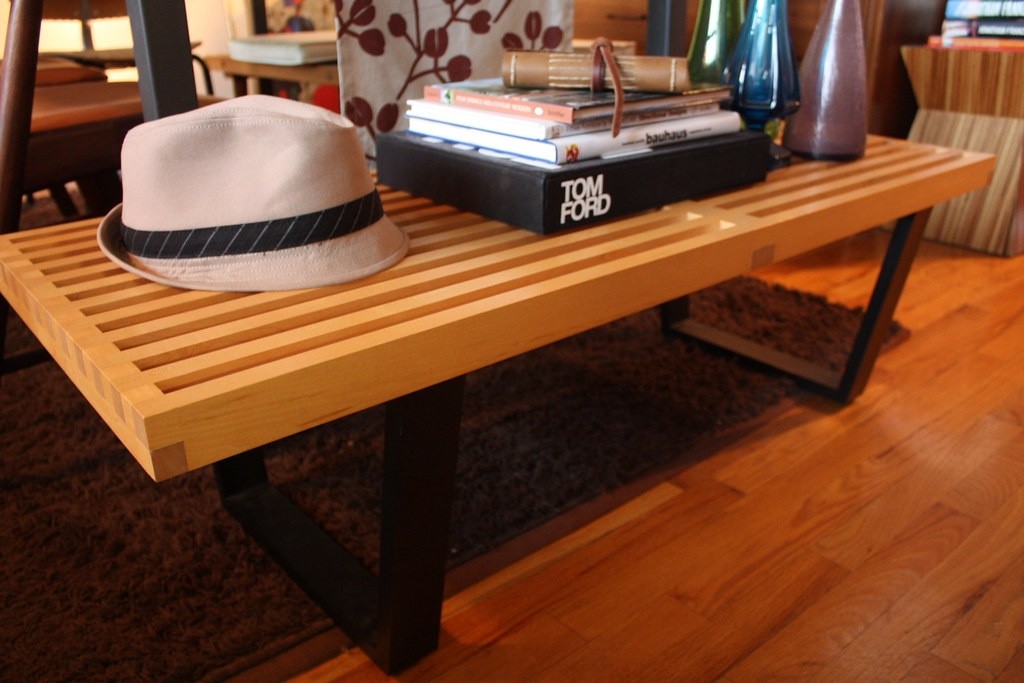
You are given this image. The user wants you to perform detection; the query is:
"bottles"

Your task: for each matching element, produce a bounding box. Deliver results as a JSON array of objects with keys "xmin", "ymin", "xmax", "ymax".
[
  {"xmin": 647, "ymin": 0, "xmax": 687, "ymax": 57},
  {"xmin": 686, "ymin": 0, "xmax": 745, "ymax": 89},
  {"xmin": 720, "ymin": 0, "xmax": 801, "ymax": 170},
  {"xmin": 787, "ymin": 0, "xmax": 868, "ymax": 160}
]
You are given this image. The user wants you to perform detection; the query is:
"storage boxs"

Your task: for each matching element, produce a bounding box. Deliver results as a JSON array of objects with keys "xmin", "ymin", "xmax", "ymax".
[{"xmin": 374, "ymin": 131, "xmax": 770, "ymax": 238}]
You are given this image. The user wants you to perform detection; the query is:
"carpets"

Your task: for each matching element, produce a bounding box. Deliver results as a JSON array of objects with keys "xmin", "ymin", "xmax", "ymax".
[{"xmin": 0, "ymin": 266, "xmax": 912, "ymax": 682}]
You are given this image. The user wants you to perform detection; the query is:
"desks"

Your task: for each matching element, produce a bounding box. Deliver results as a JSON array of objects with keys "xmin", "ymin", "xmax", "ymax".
[
  {"xmin": 204, "ymin": 54, "xmax": 340, "ymax": 99},
  {"xmin": 0, "ymin": 120, "xmax": 997, "ymax": 675}
]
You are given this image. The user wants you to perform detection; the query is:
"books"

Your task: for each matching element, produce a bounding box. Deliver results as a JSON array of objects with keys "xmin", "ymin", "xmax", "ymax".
[
  {"xmin": 937, "ymin": 1, "xmax": 1024, "ymax": 41},
  {"xmin": 226, "ymin": 29, "xmax": 337, "ymax": 67},
  {"xmin": 407, "ymin": 49, "xmax": 744, "ymax": 166}
]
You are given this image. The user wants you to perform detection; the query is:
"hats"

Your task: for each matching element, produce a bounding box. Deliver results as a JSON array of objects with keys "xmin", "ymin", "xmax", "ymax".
[{"xmin": 96, "ymin": 92, "xmax": 410, "ymax": 292}]
